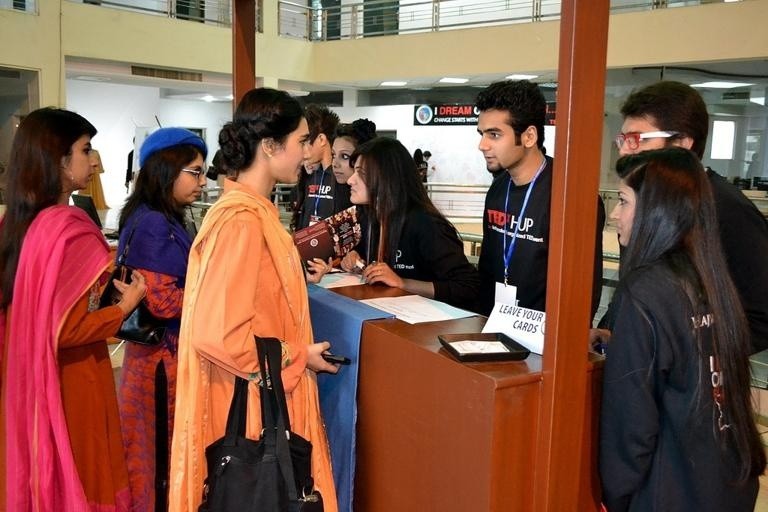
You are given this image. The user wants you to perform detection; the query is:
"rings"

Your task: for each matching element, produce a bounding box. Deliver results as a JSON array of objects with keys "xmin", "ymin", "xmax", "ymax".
[{"xmin": 372, "ymin": 260, "xmax": 378, "ymax": 266}]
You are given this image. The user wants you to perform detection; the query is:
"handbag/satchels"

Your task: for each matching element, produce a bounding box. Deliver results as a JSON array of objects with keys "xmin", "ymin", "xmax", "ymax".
[
  {"xmin": 98, "ymin": 212, "xmax": 167, "ymax": 345},
  {"xmin": 198, "ymin": 334, "xmax": 326, "ymax": 512}
]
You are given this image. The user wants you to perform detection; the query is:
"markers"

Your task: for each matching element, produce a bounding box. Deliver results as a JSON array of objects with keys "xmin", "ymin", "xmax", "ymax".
[{"xmin": 355, "ymin": 259, "xmax": 366, "ymax": 270}]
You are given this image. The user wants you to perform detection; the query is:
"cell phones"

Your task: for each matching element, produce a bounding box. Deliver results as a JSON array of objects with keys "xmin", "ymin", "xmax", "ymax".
[{"xmin": 320, "ymin": 352, "xmax": 351, "ymax": 367}]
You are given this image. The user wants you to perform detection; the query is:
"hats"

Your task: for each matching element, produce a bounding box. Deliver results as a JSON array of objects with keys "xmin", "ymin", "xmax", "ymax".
[{"xmin": 139, "ymin": 126, "xmax": 208, "ymax": 166}]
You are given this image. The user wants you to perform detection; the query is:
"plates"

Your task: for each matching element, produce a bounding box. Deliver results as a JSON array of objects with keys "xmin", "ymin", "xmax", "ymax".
[{"xmin": 438, "ymin": 332, "xmax": 531, "ymax": 362}]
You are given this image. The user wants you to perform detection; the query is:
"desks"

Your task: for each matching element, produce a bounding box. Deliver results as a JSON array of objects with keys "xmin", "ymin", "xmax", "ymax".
[{"xmin": 306, "ymin": 274, "xmax": 606, "ymax": 511}]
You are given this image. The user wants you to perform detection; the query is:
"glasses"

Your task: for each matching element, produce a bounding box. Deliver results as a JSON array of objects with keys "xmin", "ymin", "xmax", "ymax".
[
  {"xmin": 182, "ymin": 167, "xmax": 204, "ymax": 179},
  {"xmin": 615, "ymin": 130, "xmax": 679, "ymax": 150}
]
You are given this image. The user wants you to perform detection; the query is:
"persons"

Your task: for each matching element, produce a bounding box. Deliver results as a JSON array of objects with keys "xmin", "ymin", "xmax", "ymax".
[
  {"xmin": 297, "ymin": 103, "xmax": 341, "ymax": 238},
  {"xmin": 338, "ymin": 135, "xmax": 488, "ymax": 315},
  {"xmin": 302, "ymin": 160, "xmax": 321, "ymax": 174},
  {"xmin": 413, "ymin": 150, "xmax": 427, "ymax": 184},
  {"xmin": 326, "ymin": 118, "xmax": 375, "ymax": 186},
  {"xmin": 112, "ymin": 127, "xmax": 209, "ymax": 512},
  {"xmin": 177, "ymin": 88, "xmax": 342, "ymax": 512},
  {"xmin": 597, "ymin": 147, "xmax": 766, "ymax": 512},
  {"xmin": 588, "ymin": 80, "xmax": 768, "ymax": 357},
  {"xmin": 423, "ymin": 151, "xmax": 436, "ymax": 177},
  {"xmin": 0, "ymin": 107, "xmax": 155, "ymax": 512},
  {"xmin": 474, "ymin": 79, "xmax": 605, "ymax": 328}
]
